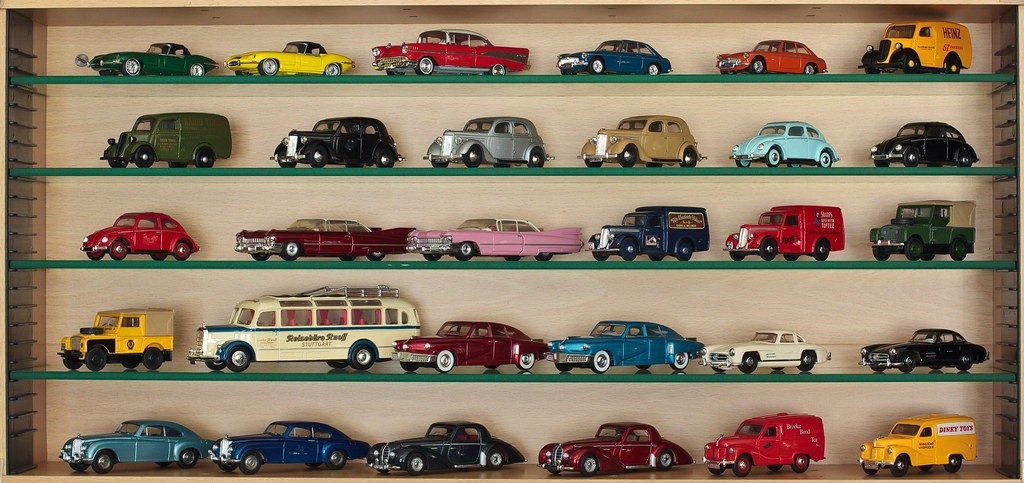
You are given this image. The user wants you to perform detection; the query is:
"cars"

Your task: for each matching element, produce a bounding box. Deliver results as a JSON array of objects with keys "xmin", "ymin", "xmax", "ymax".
[
  {"xmin": 58, "ymin": 419, "xmax": 213, "ymax": 475},
  {"xmin": 869, "ymin": 120, "xmax": 980, "ymax": 167},
  {"xmin": 390, "ymin": 319, "xmax": 550, "ymax": 372},
  {"xmin": 857, "ymin": 328, "xmax": 989, "ymax": 372},
  {"xmin": 422, "ymin": 115, "xmax": 555, "ymax": 168},
  {"xmin": 208, "ymin": 420, "xmax": 372, "ymax": 475},
  {"xmin": 556, "ymin": 38, "xmax": 674, "ymax": 76},
  {"xmin": 233, "ymin": 217, "xmax": 412, "ymax": 261},
  {"xmin": 715, "ymin": 39, "xmax": 829, "ymax": 75},
  {"xmin": 270, "ymin": 115, "xmax": 406, "ymax": 169},
  {"xmin": 543, "ymin": 319, "xmax": 706, "ymax": 374},
  {"xmin": 729, "ymin": 121, "xmax": 842, "ymax": 167},
  {"xmin": 697, "ymin": 330, "xmax": 832, "ymax": 374},
  {"xmin": 577, "ymin": 114, "xmax": 707, "ymax": 167},
  {"xmin": 405, "ymin": 216, "xmax": 584, "ymax": 261},
  {"xmin": 537, "ymin": 421, "xmax": 696, "ymax": 476},
  {"xmin": 365, "ymin": 419, "xmax": 528, "ymax": 475},
  {"xmin": 79, "ymin": 212, "xmax": 200, "ymax": 260},
  {"xmin": 369, "ymin": 28, "xmax": 532, "ymax": 76}
]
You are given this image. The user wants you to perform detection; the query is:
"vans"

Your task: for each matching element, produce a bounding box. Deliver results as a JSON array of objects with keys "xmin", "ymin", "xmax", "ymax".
[
  {"xmin": 722, "ymin": 204, "xmax": 845, "ymax": 260},
  {"xmin": 584, "ymin": 205, "xmax": 710, "ymax": 261},
  {"xmin": 857, "ymin": 19, "xmax": 973, "ymax": 75},
  {"xmin": 702, "ymin": 412, "xmax": 826, "ymax": 477},
  {"xmin": 98, "ymin": 111, "xmax": 232, "ymax": 167},
  {"xmin": 858, "ymin": 411, "xmax": 978, "ymax": 478}
]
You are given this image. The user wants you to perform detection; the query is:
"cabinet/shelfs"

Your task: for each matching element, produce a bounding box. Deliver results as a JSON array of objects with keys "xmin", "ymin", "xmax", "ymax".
[{"xmin": 3, "ymin": 0, "xmax": 1022, "ymax": 482}]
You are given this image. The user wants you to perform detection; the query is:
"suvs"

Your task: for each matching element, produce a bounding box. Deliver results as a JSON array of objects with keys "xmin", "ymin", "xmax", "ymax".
[
  {"xmin": 56, "ymin": 308, "xmax": 173, "ymax": 370},
  {"xmin": 866, "ymin": 199, "xmax": 976, "ymax": 260}
]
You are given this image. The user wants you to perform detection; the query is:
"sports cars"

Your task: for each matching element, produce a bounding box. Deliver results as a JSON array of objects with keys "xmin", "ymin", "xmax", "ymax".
[
  {"xmin": 75, "ymin": 41, "xmax": 218, "ymax": 77},
  {"xmin": 223, "ymin": 40, "xmax": 355, "ymax": 76}
]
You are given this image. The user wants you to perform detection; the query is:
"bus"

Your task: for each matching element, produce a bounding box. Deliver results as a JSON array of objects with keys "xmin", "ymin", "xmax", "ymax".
[{"xmin": 185, "ymin": 283, "xmax": 420, "ymax": 372}]
